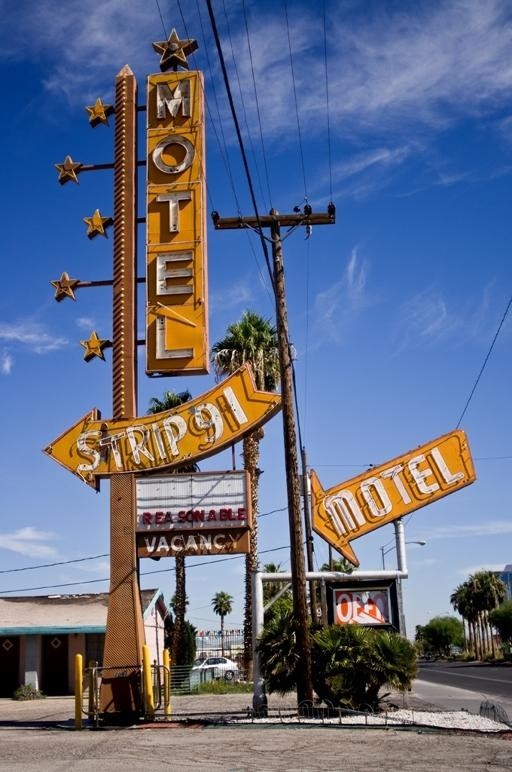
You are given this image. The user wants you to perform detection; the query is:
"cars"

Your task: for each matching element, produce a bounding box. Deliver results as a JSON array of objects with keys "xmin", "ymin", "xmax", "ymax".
[{"xmin": 192, "ymin": 657, "xmax": 238, "ymax": 681}]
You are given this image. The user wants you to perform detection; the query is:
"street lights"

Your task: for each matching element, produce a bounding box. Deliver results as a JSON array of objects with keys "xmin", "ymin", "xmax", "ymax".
[{"xmin": 382, "ymin": 541, "xmax": 425, "ymax": 570}]
